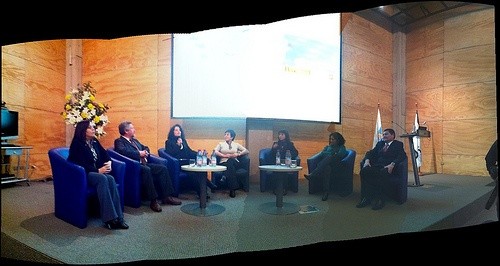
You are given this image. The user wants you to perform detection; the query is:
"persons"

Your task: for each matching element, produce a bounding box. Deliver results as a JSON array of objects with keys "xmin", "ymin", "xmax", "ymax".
[
  {"xmin": 211, "ymin": 130, "xmax": 249, "ymax": 198},
  {"xmin": 68, "ymin": 120, "xmax": 129, "ymax": 230},
  {"xmin": 166, "ymin": 125, "xmax": 216, "ymax": 200},
  {"xmin": 304, "ymin": 132, "xmax": 348, "ymax": 200},
  {"xmin": 357, "ymin": 128, "xmax": 404, "ymax": 210},
  {"xmin": 272, "ymin": 130, "xmax": 298, "ymax": 195},
  {"xmin": 114, "ymin": 121, "xmax": 182, "ymax": 210}
]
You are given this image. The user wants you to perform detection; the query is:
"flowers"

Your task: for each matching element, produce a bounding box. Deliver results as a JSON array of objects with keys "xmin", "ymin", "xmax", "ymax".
[{"xmin": 61, "ymin": 81, "xmax": 111, "ymax": 140}]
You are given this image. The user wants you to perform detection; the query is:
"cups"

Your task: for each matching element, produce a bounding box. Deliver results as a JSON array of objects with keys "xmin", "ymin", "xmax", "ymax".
[{"xmin": 189, "ymin": 159, "xmax": 195, "ymax": 167}]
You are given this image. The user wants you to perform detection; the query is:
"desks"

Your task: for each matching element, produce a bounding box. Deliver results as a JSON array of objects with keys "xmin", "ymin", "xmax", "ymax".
[
  {"xmin": 181, "ymin": 164, "xmax": 227, "ymax": 217},
  {"xmin": 258, "ymin": 165, "xmax": 304, "ymax": 215}
]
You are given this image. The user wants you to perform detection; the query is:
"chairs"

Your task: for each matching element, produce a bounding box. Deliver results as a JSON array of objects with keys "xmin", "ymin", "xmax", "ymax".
[
  {"xmin": 307, "ymin": 149, "xmax": 355, "ymax": 195},
  {"xmin": 107, "ymin": 146, "xmax": 167, "ymax": 208},
  {"xmin": 48, "ymin": 148, "xmax": 126, "ymax": 229},
  {"xmin": 259, "ymin": 148, "xmax": 301, "ymax": 193},
  {"xmin": 211, "ymin": 152, "xmax": 250, "ymax": 192},
  {"xmin": 159, "ymin": 149, "xmax": 209, "ymax": 194},
  {"xmin": 361, "ymin": 156, "xmax": 408, "ymax": 204}
]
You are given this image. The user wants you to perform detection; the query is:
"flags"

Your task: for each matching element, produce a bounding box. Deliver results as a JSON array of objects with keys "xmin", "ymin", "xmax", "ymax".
[
  {"xmin": 373, "ymin": 109, "xmax": 383, "ymax": 148},
  {"xmin": 412, "ymin": 113, "xmax": 422, "ymax": 168}
]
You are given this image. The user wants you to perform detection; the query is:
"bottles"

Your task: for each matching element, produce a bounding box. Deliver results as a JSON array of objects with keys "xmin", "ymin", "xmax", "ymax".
[
  {"xmin": 196, "ymin": 149, "xmax": 202, "ymax": 167},
  {"xmin": 202, "ymin": 150, "xmax": 207, "ymax": 167},
  {"xmin": 285, "ymin": 150, "xmax": 291, "ymax": 167},
  {"xmin": 276, "ymin": 150, "xmax": 280, "ymax": 165},
  {"xmin": 211, "ymin": 150, "xmax": 216, "ymax": 167}
]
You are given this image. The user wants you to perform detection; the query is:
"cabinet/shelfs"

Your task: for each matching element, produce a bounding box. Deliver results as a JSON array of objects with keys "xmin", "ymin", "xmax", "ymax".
[{"xmin": 1, "ymin": 143, "xmax": 33, "ymax": 186}]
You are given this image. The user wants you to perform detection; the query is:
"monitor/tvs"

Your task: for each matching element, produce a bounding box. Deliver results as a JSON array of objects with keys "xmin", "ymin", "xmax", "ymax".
[{"xmin": 1, "ymin": 111, "xmax": 19, "ymax": 146}]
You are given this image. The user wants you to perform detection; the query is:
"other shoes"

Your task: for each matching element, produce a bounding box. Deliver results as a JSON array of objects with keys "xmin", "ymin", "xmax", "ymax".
[
  {"xmin": 209, "ymin": 182, "xmax": 218, "ymax": 190},
  {"xmin": 229, "ymin": 191, "xmax": 236, "ymax": 197},
  {"xmin": 210, "ymin": 189, "xmax": 216, "ymax": 193},
  {"xmin": 356, "ymin": 198, "xmax": 372, "ymax": 207},
  {"xmin": 165, "ymin": 197, "xmax": 182, "ymax": 205},
  {"xmin": 304, "ymin": 173, "xmax": 311, "ymax": 180},
  {"xmin": 322, "ymin": 192, "xmax": 328, "ymax": 201},
  {"xmin": 106, "ymin": 218, "xmax": 129, "ymax": 230},
  {"xmin": 151, "ymin": 202, "xmax": 162, "ymax": 213},
  {"xmin": 372, "ymin": 199, "xmax": 384, "ymax": 209}
]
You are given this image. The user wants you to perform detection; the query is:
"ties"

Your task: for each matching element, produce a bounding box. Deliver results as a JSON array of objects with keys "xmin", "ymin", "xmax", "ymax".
[
  {"xmin": 129, "ymin": 139, "xmax": 146, "ymax": 164},
  {"xmin": 383, "ymin": 144, "xmax": 389, "ymax": 152}
]
